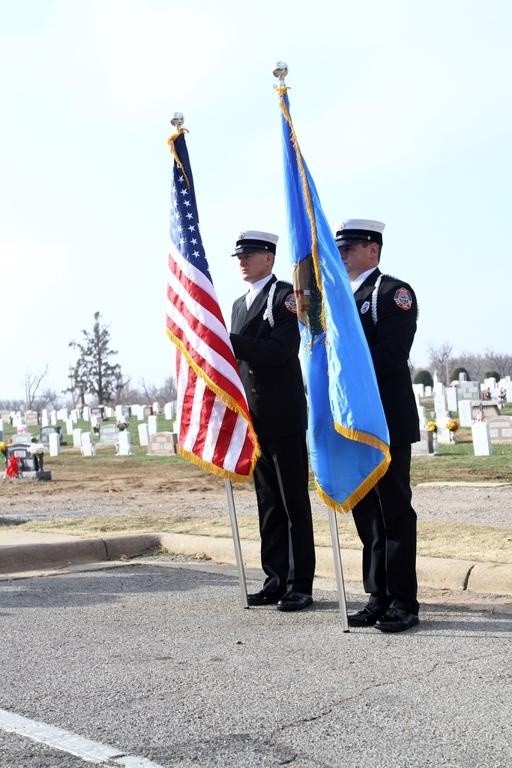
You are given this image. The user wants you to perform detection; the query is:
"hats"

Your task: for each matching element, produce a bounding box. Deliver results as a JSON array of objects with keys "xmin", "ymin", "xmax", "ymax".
[
  {"xmin": 231, "ymin": 231, "xmax": 279, "ymax": 256},
  {"xmin": 335, "ymin": 219, "xmax": 385, "ymax": 247}
]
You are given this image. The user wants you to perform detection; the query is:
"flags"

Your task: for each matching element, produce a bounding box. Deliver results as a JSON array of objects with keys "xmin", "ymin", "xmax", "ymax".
[
  {"xmin": 163, "ymin": 129, "xmax": 261, "ymax": 481},
  {"xmin": 274, "ymin": 83, "xmax": 392, "ymax": 514}
]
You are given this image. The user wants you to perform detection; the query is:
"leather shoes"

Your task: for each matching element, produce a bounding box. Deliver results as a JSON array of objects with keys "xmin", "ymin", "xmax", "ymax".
[
  {"xmin": 277, "ymin": 592, "xmax": 313, "ymax": 611},
  {"xmin": 248, "ymin": 590, "xmax": 287, "ymax": 605},
  {"xmin": 374, "ymin": 608, "xmax": 419, "ymax": 631},
  {"xmin": 348, "ymin": 603, "xmax": 389, "ymax": 626}
]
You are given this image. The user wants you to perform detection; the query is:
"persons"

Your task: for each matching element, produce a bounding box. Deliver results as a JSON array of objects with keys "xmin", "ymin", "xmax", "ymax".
[
  {"xmin": 334, "ymin": 218, "xmax": 420, "ymax": 632},
  {"xmin": 227, "ymin": 230, "xmax": 314, "ymax": 610}
]
